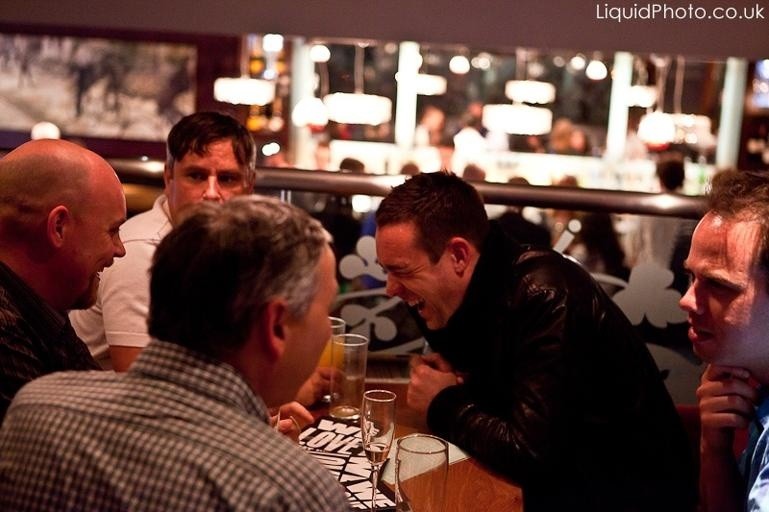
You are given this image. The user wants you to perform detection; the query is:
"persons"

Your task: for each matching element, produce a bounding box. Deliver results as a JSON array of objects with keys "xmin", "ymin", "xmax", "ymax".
[{"xmin": 0, "ymin": 111, "xmax": 769, "ymax": 512}]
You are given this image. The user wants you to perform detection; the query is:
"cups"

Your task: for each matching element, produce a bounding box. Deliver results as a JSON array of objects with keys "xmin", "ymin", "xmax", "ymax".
[
  {"xmin": 399, "ymin": 435, "xmax": 447, "ymax": 510},
  {"xmin": 318, "ymin": 318, "xmax": 368, "ymax": 422}
]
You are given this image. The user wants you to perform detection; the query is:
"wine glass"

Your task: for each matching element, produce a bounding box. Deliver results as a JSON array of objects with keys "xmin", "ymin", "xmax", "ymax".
[{"xmin": 362, "ymin": 390, "xmax": 395, "ymax": 508}]
